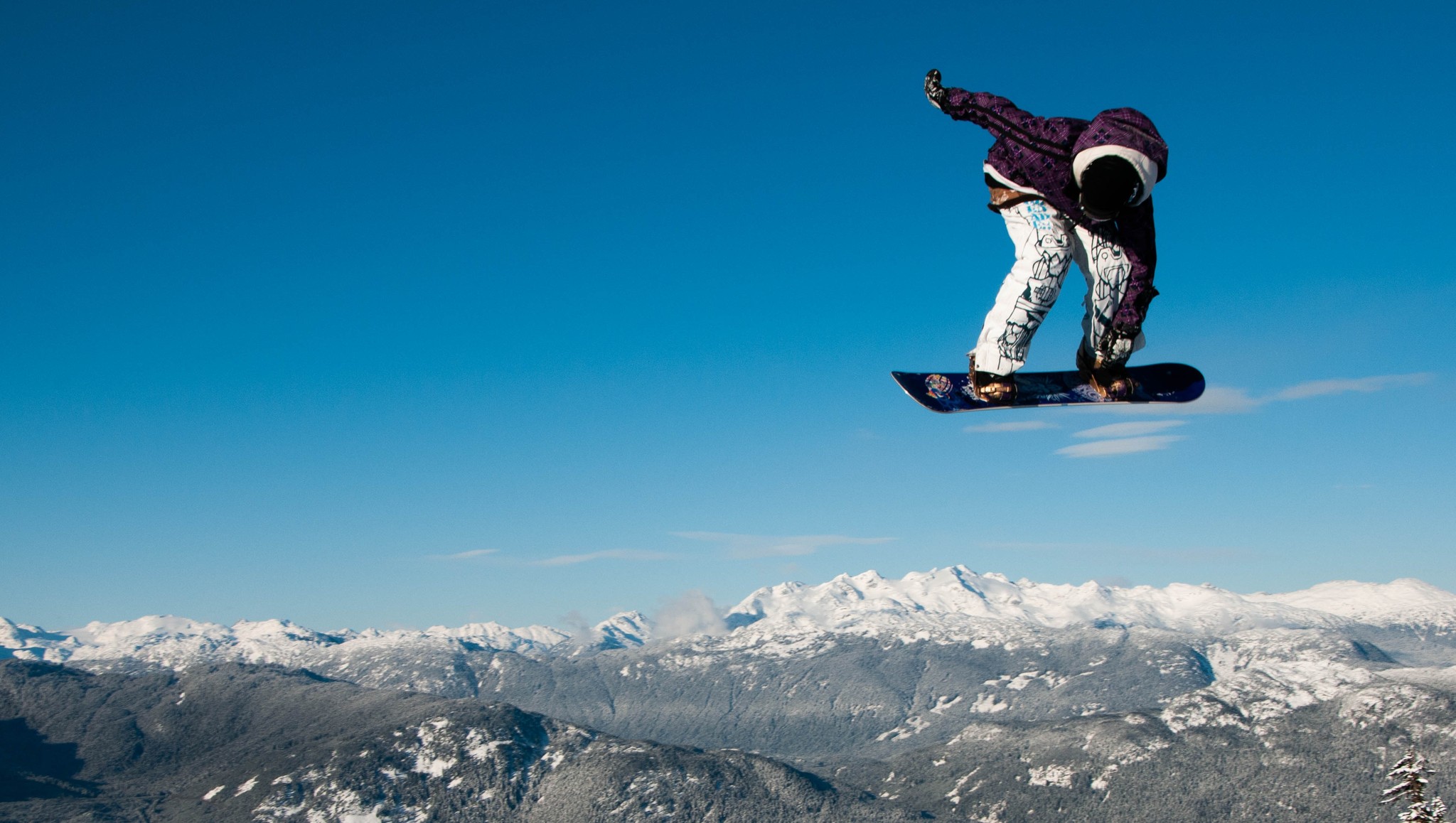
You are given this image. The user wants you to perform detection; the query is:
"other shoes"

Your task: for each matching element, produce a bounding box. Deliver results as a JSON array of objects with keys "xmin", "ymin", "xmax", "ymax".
[
  {"xmin": 982, "ymin": 373, "xmax": 1012, "ymax": 400},
  {"xmin": 1097, "ymin": 366, "xmax": 1136, "ymax": 398}
]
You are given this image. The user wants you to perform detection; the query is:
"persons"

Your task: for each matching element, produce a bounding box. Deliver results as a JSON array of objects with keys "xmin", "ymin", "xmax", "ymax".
[{"xmin": 925, "ymin": 67, "xmax": 1168, "ymax": 406}]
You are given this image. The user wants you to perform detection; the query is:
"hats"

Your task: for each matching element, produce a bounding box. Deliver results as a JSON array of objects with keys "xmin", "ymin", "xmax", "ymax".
[{"xmin": 1081, "ymin": 155, "xmax": 1143, "ymax": 218}]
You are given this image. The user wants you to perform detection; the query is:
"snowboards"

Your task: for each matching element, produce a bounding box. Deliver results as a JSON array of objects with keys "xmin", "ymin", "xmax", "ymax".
[{"xmin": 891, "ymin": 363, "xmax": 1206, "ymax": 414}]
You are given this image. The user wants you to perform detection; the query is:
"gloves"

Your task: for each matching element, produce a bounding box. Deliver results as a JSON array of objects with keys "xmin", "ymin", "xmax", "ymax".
[
  {"xmin": 924, "ymin": 69, "xmax": 945, "ymax": 110},
  {"xmin": 1095, "ymin": 326, "xmax": 1134, "ymax": 368}
]
